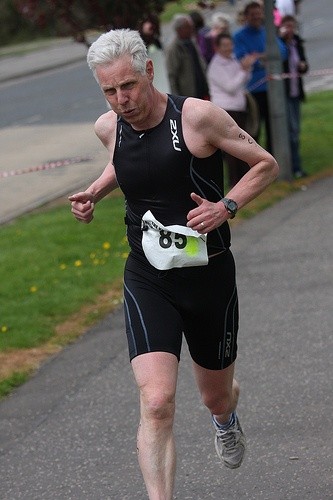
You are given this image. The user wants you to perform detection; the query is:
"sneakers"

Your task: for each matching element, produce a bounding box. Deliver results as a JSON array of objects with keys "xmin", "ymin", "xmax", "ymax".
[{"xmin": 211, "ymin": 411, "xmax": 248, "ymax": 469}]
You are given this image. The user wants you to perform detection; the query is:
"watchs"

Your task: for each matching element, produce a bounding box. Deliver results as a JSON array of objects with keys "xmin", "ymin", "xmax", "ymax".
[{"xmin": 220, "ymin": 198, "xmax": 238, "ymax": 219}]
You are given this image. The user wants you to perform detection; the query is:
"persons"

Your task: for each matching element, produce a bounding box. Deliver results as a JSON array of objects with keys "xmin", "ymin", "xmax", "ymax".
[
  {"xmin": 69, "ymin": 28, "xmax": 280, "ymax": 500},
  {"xmin": 137, "ymin": 0, "xmax": 309, "ymax": 187}
]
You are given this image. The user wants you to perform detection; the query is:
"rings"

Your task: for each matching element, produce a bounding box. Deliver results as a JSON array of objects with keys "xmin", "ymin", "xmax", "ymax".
[{"xmin": 201, "ymin": 222, "xmax": 205, "ymax": 229}]
